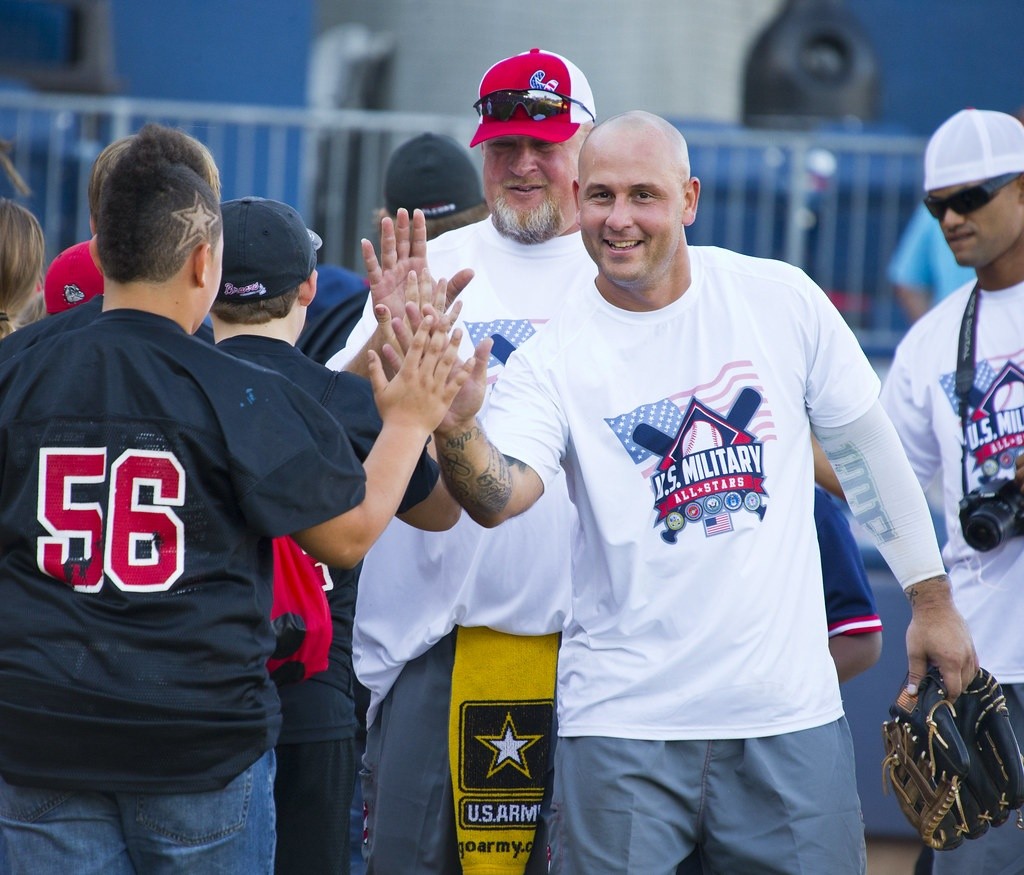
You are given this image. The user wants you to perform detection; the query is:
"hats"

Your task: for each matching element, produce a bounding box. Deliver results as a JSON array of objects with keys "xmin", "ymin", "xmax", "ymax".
[
  {"xmin": 220, "ymin": 195, "xmax": 323, "ymax": 304},
  {"xmin": 44, "ymin": 240, "xmax": 104, "ymax": 314},
  {"xmin": 924, "ymin": 109, "xmax": 1024, "ymax": 191},
  {"xmin": 470, "ymin": 49, "xmax": 595, "ymax": 148},
  {"xmin": 386, "ymin": 132, "xmax": 482, "ymax": 219}
]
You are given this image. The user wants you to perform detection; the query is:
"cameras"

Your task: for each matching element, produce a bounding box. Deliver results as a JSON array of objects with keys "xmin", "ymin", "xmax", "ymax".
[{"xmin": 959, "ymin": 477, "xmax": 1024, "ymax": 553}]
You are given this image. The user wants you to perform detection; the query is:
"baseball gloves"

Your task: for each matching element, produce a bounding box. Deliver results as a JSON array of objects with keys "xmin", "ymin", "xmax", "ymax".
[{"xmin": 881, "ymin": 664, "xmax": 1024, "ymax": 852}]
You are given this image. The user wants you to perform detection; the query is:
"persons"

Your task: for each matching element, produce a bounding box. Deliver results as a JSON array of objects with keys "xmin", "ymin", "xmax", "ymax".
[
  {"xmin": 0, "ymin": 128, "xmax": 476, "ymax": 874},
  {"xmin": 299, "ymin": 46, "xmax": 1024, "ymax": 875}
]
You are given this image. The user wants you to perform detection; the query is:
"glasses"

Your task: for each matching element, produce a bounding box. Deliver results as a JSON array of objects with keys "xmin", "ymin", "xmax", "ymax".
[
  {"xmin": 473, "ymin": 89, "xmax": 595, "ymax": 121},
  {"xmin": 924, "ymin": 173, "xmax": 1022, "ymax": 218}
]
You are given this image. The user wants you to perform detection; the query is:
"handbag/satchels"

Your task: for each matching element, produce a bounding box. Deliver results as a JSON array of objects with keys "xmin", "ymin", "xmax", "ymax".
[{"xmin": 266, "ymin": 528, "xmax": 334, "ymax": 685}]
